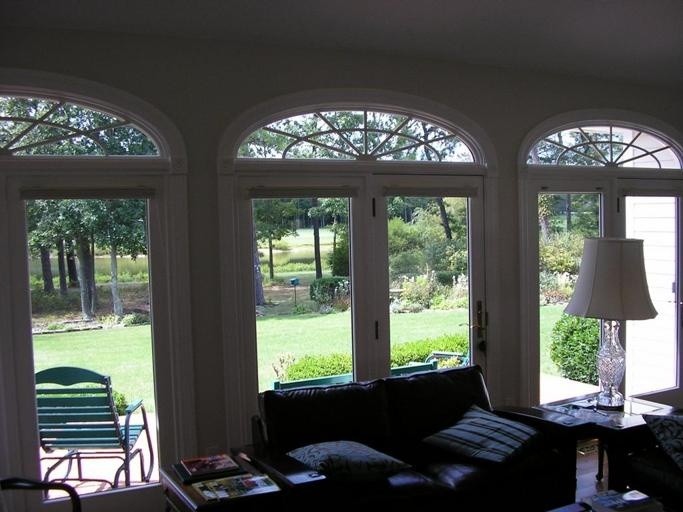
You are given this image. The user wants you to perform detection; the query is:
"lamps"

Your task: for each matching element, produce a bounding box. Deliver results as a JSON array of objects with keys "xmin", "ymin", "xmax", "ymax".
[{"xmin": 562, "ymin": 236, "xmax": 659, "ymax": 411}]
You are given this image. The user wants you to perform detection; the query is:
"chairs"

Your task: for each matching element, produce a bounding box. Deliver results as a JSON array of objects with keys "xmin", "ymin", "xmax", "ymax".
[
  {"xmin": 422, "ymin": 348, "xmax": 470, "ymax": 372},
  {"xmin": 32, "ymin": 368, "xmax": 155, "ymax": 501},
  {"xmin": 0, "ymin": 477, "xmax": 81, "ymax": 512}
]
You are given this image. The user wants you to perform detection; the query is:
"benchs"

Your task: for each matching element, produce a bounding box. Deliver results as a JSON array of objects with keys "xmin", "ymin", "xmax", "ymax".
[{"xmin": 273, "ymin": 353, "xmax": 439, "ymax": 390}]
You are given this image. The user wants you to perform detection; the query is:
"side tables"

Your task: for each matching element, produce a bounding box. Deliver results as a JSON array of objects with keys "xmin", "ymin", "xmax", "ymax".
[
  {"xmin": 544, "ymin": 392, "xmax": 675, "ymax": 480},
  {"xmin": 159, "ymin": 453, "xmax": 292, "ymax": 511}
]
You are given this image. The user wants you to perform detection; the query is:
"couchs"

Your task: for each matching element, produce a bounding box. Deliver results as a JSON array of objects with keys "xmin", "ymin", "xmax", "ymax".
[
  {"xmin": 231, "ymin": 365, "xmax": 599, "ymax": 511},
  {"xmin": 604, "ymin": 404, "xmax": 682, "ymax": 511}
]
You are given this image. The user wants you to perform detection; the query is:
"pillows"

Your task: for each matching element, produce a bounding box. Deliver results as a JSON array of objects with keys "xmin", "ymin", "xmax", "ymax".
[
  {"xmin": 420, "ymin": 405, "xmax": 540, "ymax": 464},
  {"xmin": 641, "ymin": 413, "xmax": 683, "ymax": 471},
  {"xmin": 282, "ymin": 436, "xmax": 412, "ymax": 477}
]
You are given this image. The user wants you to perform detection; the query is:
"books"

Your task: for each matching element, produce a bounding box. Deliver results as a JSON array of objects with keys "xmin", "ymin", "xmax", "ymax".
[
  {"xmin": 590, "ymin": 486, "xmax": 663, "ymax": 512},
  {"xmin": 170, "ymin": 454, "xmax": 282, "ymax": 504}
]
